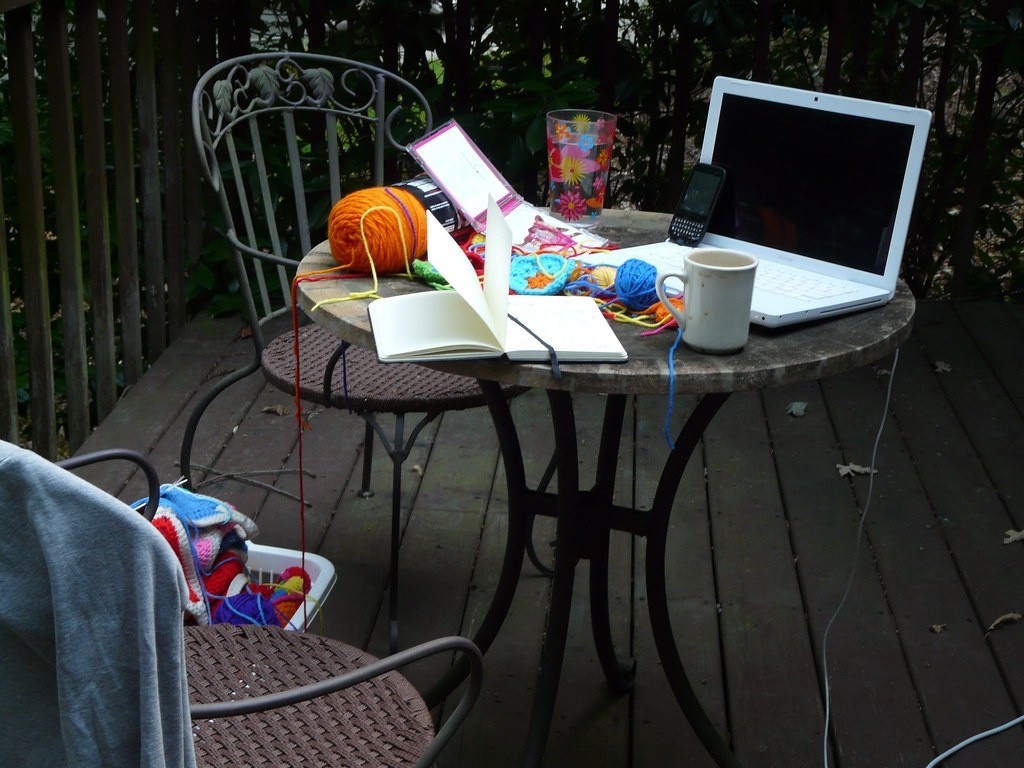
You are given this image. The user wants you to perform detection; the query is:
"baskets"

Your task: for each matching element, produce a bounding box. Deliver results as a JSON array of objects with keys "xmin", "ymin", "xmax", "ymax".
[{"xmin": 192, "ymin": 538, "xmax": 337, "ymax": 739}]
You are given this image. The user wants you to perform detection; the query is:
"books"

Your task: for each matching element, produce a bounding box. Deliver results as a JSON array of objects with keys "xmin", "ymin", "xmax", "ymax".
[{"xmin": 366, "ymin": 192, "xmax": 629, "ymax": 380}]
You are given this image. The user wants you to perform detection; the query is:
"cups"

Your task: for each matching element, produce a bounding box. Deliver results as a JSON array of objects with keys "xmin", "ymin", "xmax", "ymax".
[
  {"xmin": 655, "ymin": 248, "xmax": 759, "ymax": 354},
  {"xmin": 545, "ymin": 108, "xmax": 618, "ymax": 226}
]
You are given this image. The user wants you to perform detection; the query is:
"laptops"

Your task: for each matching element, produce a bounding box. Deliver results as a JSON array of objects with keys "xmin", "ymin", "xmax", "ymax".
[{"xmin": 569, "ymin": 76, "xmax": 932, "ymax": 329}]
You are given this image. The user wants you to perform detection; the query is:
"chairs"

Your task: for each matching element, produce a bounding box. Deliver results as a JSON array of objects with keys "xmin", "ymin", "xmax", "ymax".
[
  {"xmin": 0, "ymin": 437, "xmax": 481, "ymax": 768},
  {"xmin": 181, "ymin": 51, "xmax": 552, "ymax": 661}
]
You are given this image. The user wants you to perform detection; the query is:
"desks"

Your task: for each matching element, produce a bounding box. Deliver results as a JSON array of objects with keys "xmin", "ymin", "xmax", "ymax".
[{"xmin": 294, "ymin": 208, "xmax": 917, "ymax": 768}]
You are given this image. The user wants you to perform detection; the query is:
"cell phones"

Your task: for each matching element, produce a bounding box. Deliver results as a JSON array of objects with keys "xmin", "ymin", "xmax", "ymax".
[{"xmin": 668, "ymin": 162, "xmax": 726, "ymax": 246}]
